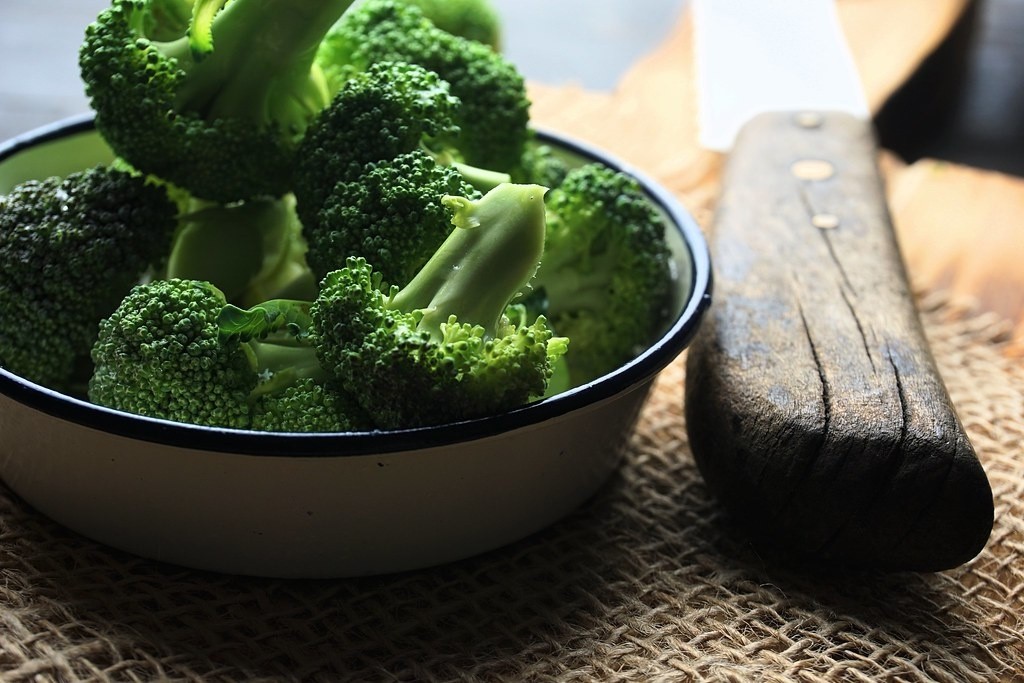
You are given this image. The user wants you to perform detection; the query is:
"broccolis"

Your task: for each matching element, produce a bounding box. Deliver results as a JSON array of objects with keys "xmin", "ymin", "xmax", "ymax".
[{"xmin": 0, "ymin": 0, "xmax": 672, "ymax": 434}]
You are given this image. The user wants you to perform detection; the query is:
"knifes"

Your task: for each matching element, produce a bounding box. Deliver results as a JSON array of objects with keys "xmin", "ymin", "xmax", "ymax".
[{"xmin": 691, "ymin": 1, "xmax": 996, "ymax": 574}]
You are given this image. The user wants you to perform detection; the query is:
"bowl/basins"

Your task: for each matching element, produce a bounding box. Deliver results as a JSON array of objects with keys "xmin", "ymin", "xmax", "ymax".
[{"xmin": 0, "ymin": 110, "xmax": 712, "ymax": 582}]
